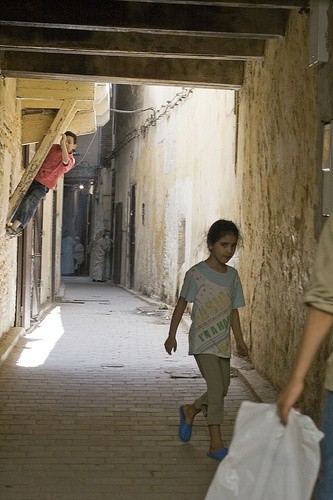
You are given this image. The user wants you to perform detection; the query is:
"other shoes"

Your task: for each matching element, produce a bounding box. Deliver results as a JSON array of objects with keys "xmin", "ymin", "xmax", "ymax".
[
  {"xmin": 14, "ymin": 226, "xmax": 21, "ymax": 237},
  {"xmin": 5, "ymin": 226, "xmax": 18, "ymax": 237}
]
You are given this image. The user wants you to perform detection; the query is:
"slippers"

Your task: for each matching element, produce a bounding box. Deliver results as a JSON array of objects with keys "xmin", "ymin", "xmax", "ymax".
[
  {"xmin": 177, "ymin": 405, "xmax": 193, "ymax": 441},
  {"xmin": 206, "ymin": 447, "xmax": 229, "ymax": 462}
]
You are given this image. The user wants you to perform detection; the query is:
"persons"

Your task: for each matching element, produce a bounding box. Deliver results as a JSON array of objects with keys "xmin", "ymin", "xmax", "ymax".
[
  {"xmin": 6, "ymin": 127, "xmax": 78, "ymax": 235},
  {"xmin": 276, "ymin": 212, "xmax": 333, "ymax": 500},
  {"xmin": 163, "ymin": 220, "xmax": 249, "ymax": 461},
  {"xmin": 61, "ymin": 229, "xmax": 115, "ymax": 283}
]
